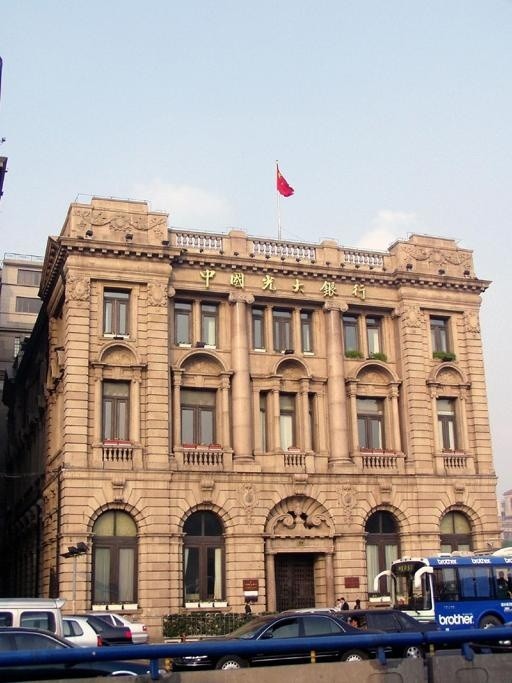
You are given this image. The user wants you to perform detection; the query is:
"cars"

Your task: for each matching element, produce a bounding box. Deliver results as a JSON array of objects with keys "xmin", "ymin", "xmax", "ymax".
[
  {"xmin": 70, "ymin": 614, "xmax": 133, "ymax": 646},
  {"xmin": 279, "ymin": 607, "xmax": 339, "ymax": 614},
  {"xmin": 20, "ymin": 616, "xmax": 104, "ymax": 648},
  {"xmin": 170, "ymin": 612, "xmax": 394, "ymax": 673},
  {"xmin": 91, "ymin": 612, "xmax": 150, "ymax": 644},
  {"xmin": 329, "ymin": 608, "xmax": 442, "ymax": 658},
  {"xmin": 0, "ymin": 626, "xmax": 168, "ymax": 682}
]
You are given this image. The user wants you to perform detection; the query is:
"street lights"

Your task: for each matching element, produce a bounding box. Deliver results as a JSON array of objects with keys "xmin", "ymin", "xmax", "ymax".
[{"xmin": 60, "ymin": 542, "xmax": 89, "ymax": 615}]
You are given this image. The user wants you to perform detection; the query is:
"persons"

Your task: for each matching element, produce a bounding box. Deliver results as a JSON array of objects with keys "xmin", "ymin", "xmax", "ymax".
[
  {"xmin": 494, "ymin": 570, "xmax": 509, "ymax": 592},
  {"xmin": 353, "ymin": 599, "xmax": 361, "ymax": 609},
  {"xmin": 336, "ymin": 598, "xmax": 342, "ymax": 609},
  {"xmin": 348, "ymin": 618, "xmax": 360, "ymax": 628},
  {"xmin": 340, "ymin": 596, "xmax": 349, "ymax": 609},
  {"xmin": 244, "ymin": 599, "xmax": 252, "ymax": 615}
]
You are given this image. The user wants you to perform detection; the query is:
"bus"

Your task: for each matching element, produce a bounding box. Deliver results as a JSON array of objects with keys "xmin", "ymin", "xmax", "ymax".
[{"xmin": 373, "ymin": 547, "xmax": 512, "ymax": 653}]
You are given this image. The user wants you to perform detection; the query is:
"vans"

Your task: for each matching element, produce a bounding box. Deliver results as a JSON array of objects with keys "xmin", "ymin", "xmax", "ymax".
[{"xmin": 0, "ymin": 597, "xmax": 64, "ymax": 637}]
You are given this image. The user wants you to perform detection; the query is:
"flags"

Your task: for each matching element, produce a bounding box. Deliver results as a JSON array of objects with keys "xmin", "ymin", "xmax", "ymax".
[{"xmin": 275, "ymin": 168, "xmax": 294, "ymax": 197}]
